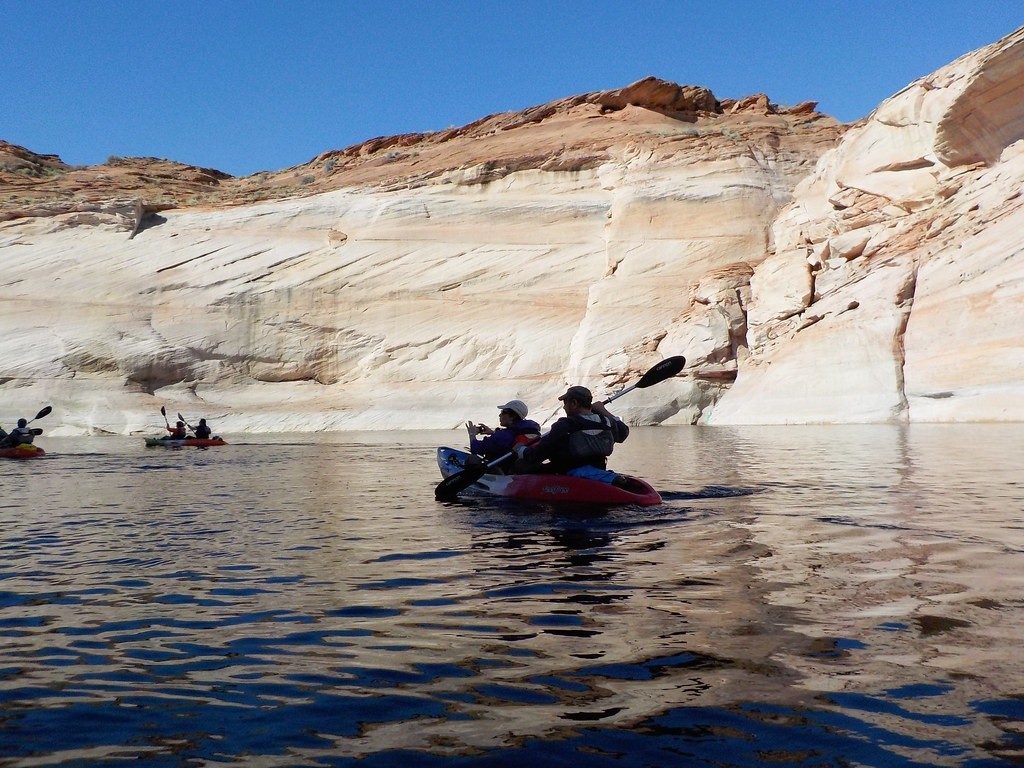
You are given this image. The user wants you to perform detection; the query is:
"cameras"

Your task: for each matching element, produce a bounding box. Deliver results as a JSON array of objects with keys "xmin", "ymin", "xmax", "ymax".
[{"xmin": 476, "ymin": 427, "xmax": 484, "ymax": 433}]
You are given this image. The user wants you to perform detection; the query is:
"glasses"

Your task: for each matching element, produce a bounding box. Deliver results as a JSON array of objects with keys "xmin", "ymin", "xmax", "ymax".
[{"xmin": 501, "ymin": 410, "xmax": 509, "ymax": 415}]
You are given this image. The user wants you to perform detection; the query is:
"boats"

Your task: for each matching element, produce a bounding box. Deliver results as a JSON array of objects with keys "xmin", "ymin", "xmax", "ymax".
[
  {"xmin": 433, "ymin": 442, "xmax": 666, "ymax": 507},
  {"xmin": 0, "ymin": 444, "xmax": 47, "ymax": 460},
  {"xmin": 141, "ymin": 436, "xmax": 229, "ymax": 447}
]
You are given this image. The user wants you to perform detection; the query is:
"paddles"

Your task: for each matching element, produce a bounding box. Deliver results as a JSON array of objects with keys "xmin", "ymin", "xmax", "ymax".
[
  {"xmin": 434, "ymin": 354, "xmax": 686, "ymax": 499},
  {"xmin": 160, "ymin": 405, "xmax": 172, "ymax": 434},
  {"xmin": 27, "ymin": 406, "xmax": 52, "ymax": 425},
  {"xmin": 177, "ymin": 412, "xmax": 195, "ymax": 436}
]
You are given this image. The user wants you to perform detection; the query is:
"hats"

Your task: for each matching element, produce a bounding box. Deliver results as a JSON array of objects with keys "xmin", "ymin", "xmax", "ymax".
[
  {"xmin": 497, "ymin": 400, "xmax": 528, "ymax": 419},
  {"xmin": 558, "ymin": 386, "xmax": 593, "ymax": 402}
]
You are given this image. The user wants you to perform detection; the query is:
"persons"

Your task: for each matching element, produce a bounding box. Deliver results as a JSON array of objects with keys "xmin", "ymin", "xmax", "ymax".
[
  {"xmin": 186, "ymin": 419, "xmax": 211, "ymax": 439},
  {"xmin": 514, "ymin": 387, "xmax": 630, "ymax": 475},
  {"xmin": 465, "ymin": 400, "xmax": 541, "ymax": 476},
  {"xmin": 153, "ymin": 421, "xmax": 186, "ymax": 440},
  {"xmin": 0, "ymin": 418, "xmax": 35, "ymax": 448}
]
